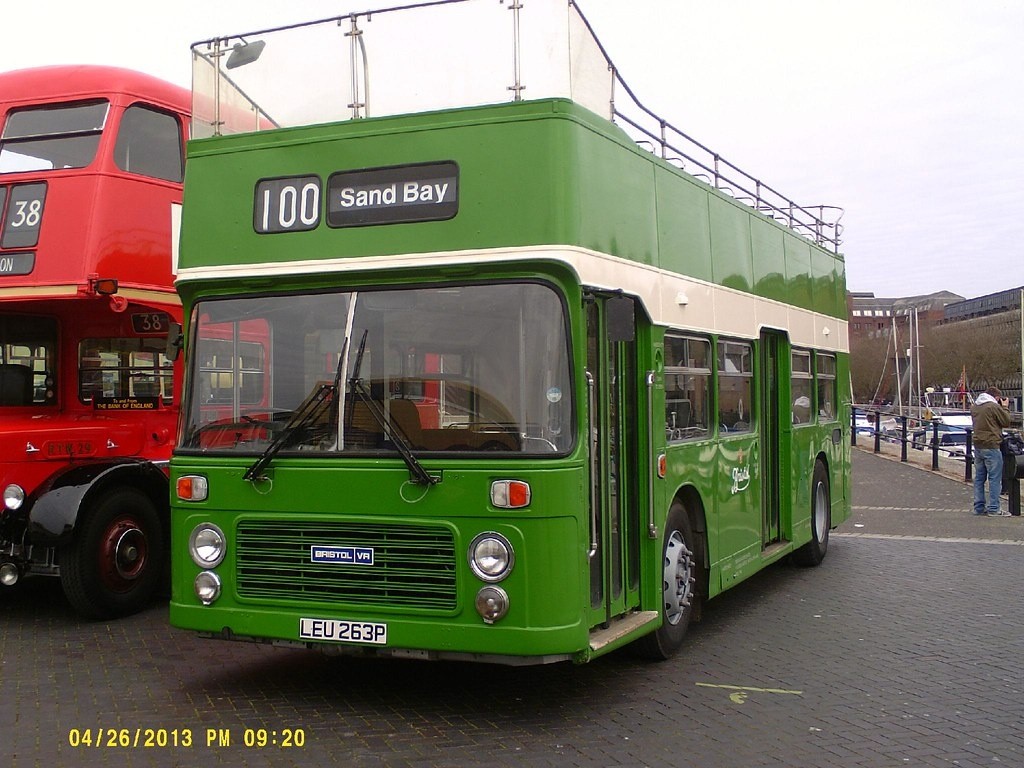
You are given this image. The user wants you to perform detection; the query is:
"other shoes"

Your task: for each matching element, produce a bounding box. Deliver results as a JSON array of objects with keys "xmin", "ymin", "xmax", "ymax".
[{"xmin": 987, "ymin": 510, "xmax": 1012, "ymax": 516}]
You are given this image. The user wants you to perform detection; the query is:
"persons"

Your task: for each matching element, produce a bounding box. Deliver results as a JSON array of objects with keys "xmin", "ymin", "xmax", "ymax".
[{"xmin": 969, "ymin": 386, "xmax": 1012, "ymax": 517}]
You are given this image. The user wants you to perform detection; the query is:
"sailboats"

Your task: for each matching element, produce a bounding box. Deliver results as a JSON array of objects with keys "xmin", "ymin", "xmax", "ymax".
[{"xmin": 851, "ymin": 307, "xmax": 977, "ymax": 464}]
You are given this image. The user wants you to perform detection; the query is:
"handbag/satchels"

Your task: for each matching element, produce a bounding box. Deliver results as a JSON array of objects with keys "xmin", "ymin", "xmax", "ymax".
[{"xmin": 999, "ymin": 432, "xmax": 1024, "ymax": 454}]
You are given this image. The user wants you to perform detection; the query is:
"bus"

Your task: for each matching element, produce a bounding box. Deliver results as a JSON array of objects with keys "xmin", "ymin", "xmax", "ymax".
[
  {"xmin": 168, "ymin": 0, "xmax": 858, "ymax": 673},
  {"xmin": 0, "ymin": 64, "xmax": 284, "ymax": 621}
]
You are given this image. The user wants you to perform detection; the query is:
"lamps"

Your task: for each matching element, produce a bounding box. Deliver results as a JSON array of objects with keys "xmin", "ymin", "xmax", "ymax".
[{"xmin": 226, "ymin": 40, "xmax": 266, "ymax": 69}]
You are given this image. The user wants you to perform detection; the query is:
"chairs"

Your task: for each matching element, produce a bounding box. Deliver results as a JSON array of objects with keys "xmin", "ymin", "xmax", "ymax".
[
  {"xmin": 51, "ymin": 136, "xmax": 183, "ymax": 183},
  {"xmin": 309, "ymin": 352, "xmax": 833, "ymax": 447},
  {"xmin": 0, "ymin": 348, "xmax": 262, "ymax": 407}
]
